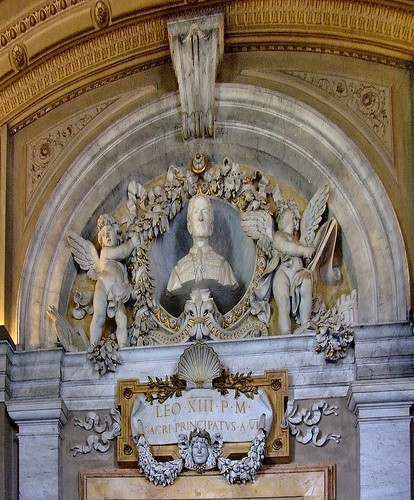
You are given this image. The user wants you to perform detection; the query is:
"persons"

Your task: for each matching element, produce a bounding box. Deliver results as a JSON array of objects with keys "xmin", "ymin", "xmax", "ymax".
[
  {"xmin": 89, "ymin": 214, "xmax": 140, "ymax": 347},
  {"xmin": 167, "ymin": 194, "xmax": 241, "ymax": 294},
  {"xmin": 271, "ymin": 199, "xmax": 315, "ymax": 334},
  {"xmin": 189, "ymin": 431, "xmax": 211, "ymax": 463}
]
